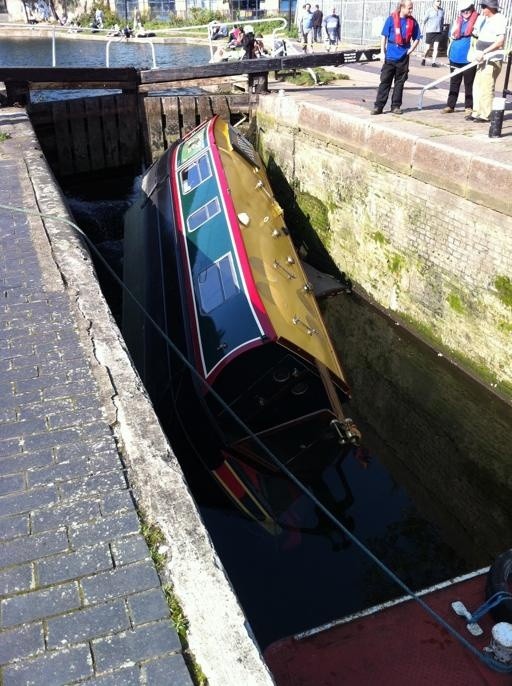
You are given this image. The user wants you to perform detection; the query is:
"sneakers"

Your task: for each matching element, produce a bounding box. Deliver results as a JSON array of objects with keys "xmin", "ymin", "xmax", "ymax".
[
  {"xmin": 440, "ymin": 106, "xmax": 490, "ymax": 123},
  {"xmin": 390, "ymin": 105, "xmax": 403, "ymax": 114},
  {"xmin": 371, "ymin": 107, "xmax": 383, "ymax": 116}
]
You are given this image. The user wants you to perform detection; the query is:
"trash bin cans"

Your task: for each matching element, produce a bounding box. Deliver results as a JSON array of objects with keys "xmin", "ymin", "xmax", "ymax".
[{"xmin": 427, "ymin": 24, "xmax": 449, "ymax": 57}]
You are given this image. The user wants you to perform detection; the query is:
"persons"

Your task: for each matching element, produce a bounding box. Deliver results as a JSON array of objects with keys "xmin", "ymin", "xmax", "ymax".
[
  {"xmin": 420, "ymin": 0, "xmax": 446, "ymax": 67},
  {"xmin": 207, "ymin": 14, "xmax": 268, "ymax": 59},
  {"xmin": 296, "ymin": 2, "xmax": 343, "ymax": 54},
  {"xmin": 369, "ymin": 0, "xmax": 421, "ymax": 115},
  {"xmin": 439, "ymin": 0, "xmax": 480, "ymax": 120},
  {"xmin": 464, "ymin": 0, "xmax": 508, "ymax": 122},
  {"xmin": 59, "ymin": 5, "xmax": 145, "ymax": 38}
]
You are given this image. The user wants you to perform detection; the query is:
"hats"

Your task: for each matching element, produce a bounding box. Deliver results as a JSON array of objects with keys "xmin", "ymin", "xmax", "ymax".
[
  {"xmin": 421, "ymin": 59, "xmax": 426, "ymax": 66},
  {"xmin": 457, "ymin": 1, "xmax": 475, "ymax": 12},
  {"xmin": 431, "ymin": 62, "xmax": 438, "ymax": 68},
  {"xmin": 479, "ymin": 0, "xmax": 503, "ymax": 10}
]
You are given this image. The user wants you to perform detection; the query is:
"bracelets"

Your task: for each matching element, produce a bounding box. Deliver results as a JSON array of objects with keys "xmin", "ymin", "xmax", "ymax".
[{"xmin": 481, "ymin": 50, "xmax": 486, "ymax": 55}]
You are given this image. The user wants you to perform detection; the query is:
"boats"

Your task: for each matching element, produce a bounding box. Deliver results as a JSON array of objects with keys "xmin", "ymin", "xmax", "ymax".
[{"xmin": 115, "ymin": 109, "xmax": 372, "ymax": 552}]
